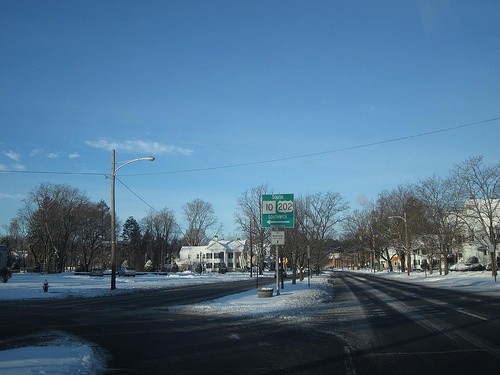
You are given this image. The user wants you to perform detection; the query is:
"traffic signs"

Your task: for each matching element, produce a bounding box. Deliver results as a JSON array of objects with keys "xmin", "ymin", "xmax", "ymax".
[{"xmin": 261, "ymin": 194, "xmax": 295, "ymax": 229}]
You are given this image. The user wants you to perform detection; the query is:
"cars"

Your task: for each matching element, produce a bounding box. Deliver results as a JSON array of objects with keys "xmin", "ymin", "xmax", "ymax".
[
  {"xmin": 274, "ymin": 268, "xmax": 287, "ymax": 278},
  {"xmin": 118, "ymin": 266, "xmax": 135, "ymax": 278},
  {"xmin": 298, "ymin": 267, "xmax": 315, "ymax": 273},
  {"xmin": 90, "ymin": 268, "xmax": 104, "ymax": 276}
]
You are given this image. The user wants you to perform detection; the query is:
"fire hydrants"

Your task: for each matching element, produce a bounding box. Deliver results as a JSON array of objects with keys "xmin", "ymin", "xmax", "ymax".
[{"xmin": 42, "ymin": 279, "xmax": 49, "ymax": 292}]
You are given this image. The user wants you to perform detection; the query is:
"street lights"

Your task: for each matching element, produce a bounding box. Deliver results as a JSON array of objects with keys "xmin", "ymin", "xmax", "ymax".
[
  {"xmin": 110, "ymin": 149, "xmax": 154, "ymax": 291},
  {"xmin": 388, "ymin": 213, "xmax": 412, "ymax": 275}
]
